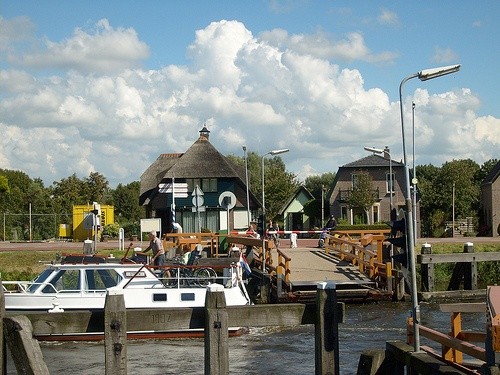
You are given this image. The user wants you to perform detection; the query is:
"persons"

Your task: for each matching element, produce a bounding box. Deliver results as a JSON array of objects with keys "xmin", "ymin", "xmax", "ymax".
[
  {"xmin": 323, "ymin": 215, "xmax": 336, "ymax": 245},
  {"xmin": 139, "ymin": 230, "xmax": 165, "ymax": 270},
  {"xmin": 168, "ymin": 222, "xmax": 184, "ymax": 238}
]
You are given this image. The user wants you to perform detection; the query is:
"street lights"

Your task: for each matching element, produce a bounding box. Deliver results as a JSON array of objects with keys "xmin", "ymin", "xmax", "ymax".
[
  {"xmin": 262, "ymin": 148, "xmax": 290, "ymax": 272},
  {"xmin": 364, "ymin": 146, "xmax": 394, "ymax": 269},
  {"xmin": 242, "ymin": 145, "xmax": 250, "ymax": 227},
  {"xmin": 399, "ymin": 63, "xmax": 462, "ymax": 351}
]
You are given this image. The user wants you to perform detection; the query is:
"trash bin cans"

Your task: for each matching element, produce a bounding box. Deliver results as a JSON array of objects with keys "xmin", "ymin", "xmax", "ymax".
[{"xmin": 218, "ymin": 230, "xmax": 228, "ymax": 253}]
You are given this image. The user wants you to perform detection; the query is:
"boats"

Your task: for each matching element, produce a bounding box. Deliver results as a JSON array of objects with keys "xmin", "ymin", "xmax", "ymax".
[{"xmin": 2, "ymin": 254, "xmax": 257, "ymax": 342}]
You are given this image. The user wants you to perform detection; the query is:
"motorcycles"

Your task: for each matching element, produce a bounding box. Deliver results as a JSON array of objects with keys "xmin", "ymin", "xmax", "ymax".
[{"xmin": 318, "ymin": 228, "xmax": 335, "ymax": 247}]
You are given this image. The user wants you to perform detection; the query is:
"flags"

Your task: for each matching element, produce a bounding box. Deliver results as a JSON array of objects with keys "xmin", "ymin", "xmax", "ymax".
[{"xmin": 239, "ymin": 252, "xmax": 251, "ymax": 276}]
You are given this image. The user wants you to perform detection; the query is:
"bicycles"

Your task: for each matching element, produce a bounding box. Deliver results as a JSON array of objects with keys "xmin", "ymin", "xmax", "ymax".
[{"xmin": 158, "ymin": 254, "xmax": 217, "ymax": 288}]
